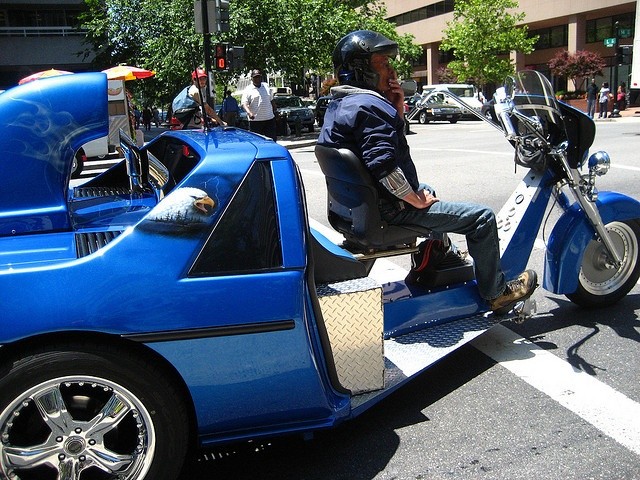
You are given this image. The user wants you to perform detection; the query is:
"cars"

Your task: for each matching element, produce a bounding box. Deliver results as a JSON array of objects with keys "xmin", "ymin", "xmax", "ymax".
[
  {"xmin": 481, "ymin": 96, "xmax": 509, "ymax": 119},
  {"xmin": 403, "ymin": 94, "xmax": 461, "ymax": 123}
]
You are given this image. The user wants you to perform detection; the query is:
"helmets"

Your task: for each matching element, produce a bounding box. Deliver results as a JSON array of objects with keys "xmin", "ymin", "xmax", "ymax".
[
  {"xmin": 251, "ymin": 70, "xmax": 262, "ymax": 77},
  {"xmin": 192, "ymin": 69, "xmax": 206, "ymax": 80},
  {"xmin": 334, "ymin": 30, "xmax": 398, "ymax": 88}
]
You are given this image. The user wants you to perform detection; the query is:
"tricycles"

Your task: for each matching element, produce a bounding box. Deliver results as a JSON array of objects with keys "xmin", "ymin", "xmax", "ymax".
[{"xmin": 0, "ymin": 68, "xmax": 639, "ymax": 479}]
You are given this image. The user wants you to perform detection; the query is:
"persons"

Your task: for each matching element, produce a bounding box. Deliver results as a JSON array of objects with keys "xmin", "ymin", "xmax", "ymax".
[
  {"xmin": 598, "ymin": 81, "xmax": 610, "ymax": 118},
  {"xmin": 172, "ymin": 67, "xmax": 228, "ymax": 129},
  {"xmin": 616, "ymin": 83, "xmax": 626, "ymax": 103},
  {"xmin": 222, "ymin": 90, "xmax": 239, "ymax": 127},
  {"xmin": 240, "ymin": 69, "xmax": 277, "ymax": 142},
  {"xmin": 586, "ymin": 77, "xmax": 598, "ymax": 118},
  {"xmin": 152, "ymin": 107, "xmax": 161, "ymax": 128},
  {"xmin": 314, "ymin": 31, "xmax": 538, "ymax": 316},
  {"xmin": 142, "ymin": 105, "xmax": 151, "ymax": 131},
  {"xmin": 132, "ymin": 106, "xmax": 141, "ymax": 130}
]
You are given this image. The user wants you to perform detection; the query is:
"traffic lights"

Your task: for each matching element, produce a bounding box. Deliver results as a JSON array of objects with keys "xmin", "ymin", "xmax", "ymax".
[
  {"xmin": 216, "ymin": 0, "xmax": 229, "ymax": 32},
  {"xmin": 215, "ymin": 42, "xmax": 228, "ymax": 70}
]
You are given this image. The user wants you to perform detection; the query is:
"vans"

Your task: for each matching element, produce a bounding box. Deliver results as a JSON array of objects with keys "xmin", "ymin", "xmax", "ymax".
[{"xmin": 316, "ymin": 95, "xmax": 331, "ymax": 125}]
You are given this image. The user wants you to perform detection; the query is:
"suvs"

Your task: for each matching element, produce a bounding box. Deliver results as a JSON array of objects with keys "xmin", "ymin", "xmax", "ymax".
[{"xmin": 271, "ymin": 96, "xmax": 314, "ymax": 132}]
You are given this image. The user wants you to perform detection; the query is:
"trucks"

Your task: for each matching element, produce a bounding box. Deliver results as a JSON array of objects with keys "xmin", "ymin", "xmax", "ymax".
[{"xmin": 422, "ymin": 83, "xmax": 482, "ymax": 120}]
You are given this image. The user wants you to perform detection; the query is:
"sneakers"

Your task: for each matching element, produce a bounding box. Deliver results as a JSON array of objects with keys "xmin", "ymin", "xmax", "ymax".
[{"xmin": 491, "ymin": 270, "xmax": 537, "ymax": 314}]
